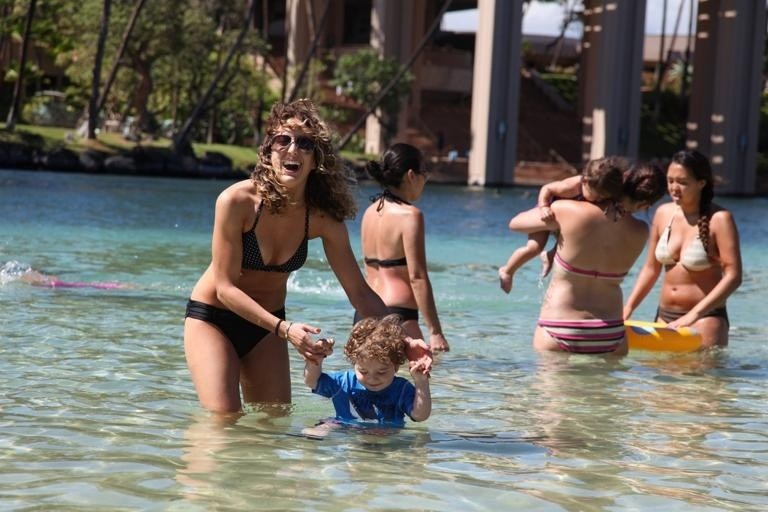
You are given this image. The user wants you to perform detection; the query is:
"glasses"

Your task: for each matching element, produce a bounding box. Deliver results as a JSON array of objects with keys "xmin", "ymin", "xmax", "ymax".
[{"xmin": 267, "ymin": 133, "xmax": 315, "ymax": 151}]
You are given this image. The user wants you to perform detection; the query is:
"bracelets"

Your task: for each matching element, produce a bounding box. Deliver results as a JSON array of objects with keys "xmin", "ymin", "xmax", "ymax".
[
  {"xmin": 275, "ymin": 318, "xmax": 284, "ymax": 337},
  {"xmin": 284, "ymin": 320, "xmax": 296, "ymax": 341}
]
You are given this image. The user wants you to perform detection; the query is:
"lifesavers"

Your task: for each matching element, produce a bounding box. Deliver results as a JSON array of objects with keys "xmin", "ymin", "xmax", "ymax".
[{"xmin": 625, "ymin": 322, "xmax": 702, "ymax": 353}]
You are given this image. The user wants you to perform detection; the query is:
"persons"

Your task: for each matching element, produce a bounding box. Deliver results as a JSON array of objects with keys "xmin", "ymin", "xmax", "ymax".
[
  {"xmin": 506, "ymin": 154, "xmax": 668, "ymax": 354},
  {"xmin": 11, "ymin": 266, "xmax": 143, "ymax": 291},
  {"xmin": 349, "ymin": 140, "xmax": 451, "ymax": 353},
  {"xmin": 496, "ymin": 154, "xmax": 628, "ymax": 294},
  {"xmin": 183, "ymin": 95, "xmax": 434, "ymax": 421},
  {"xmin": 621, "ymin": 149, "xmax": 743, "ymax": 349},
  {"xmin": 301, "ymin": 308, "xmax": 434, "ymax": 444}
]
